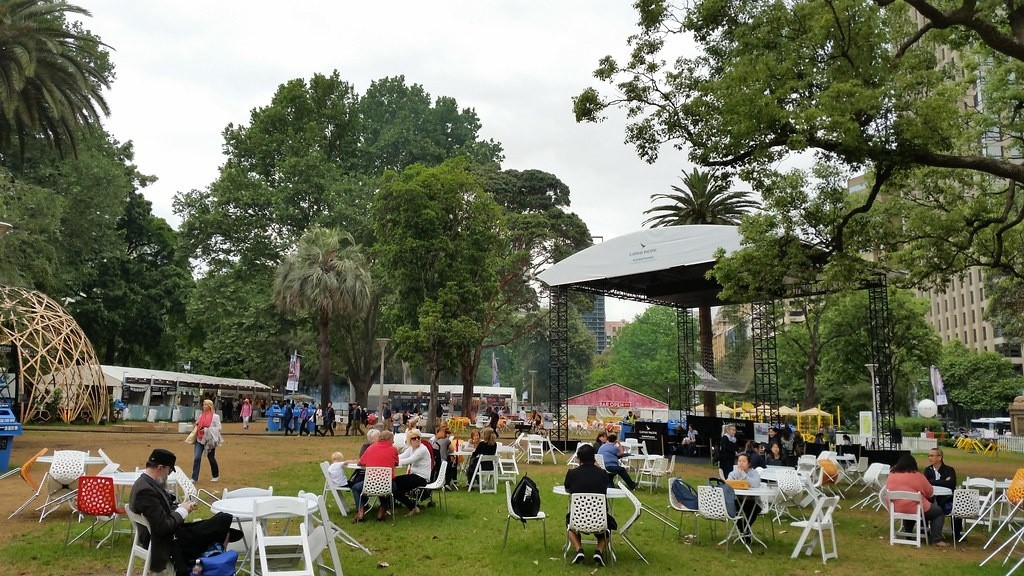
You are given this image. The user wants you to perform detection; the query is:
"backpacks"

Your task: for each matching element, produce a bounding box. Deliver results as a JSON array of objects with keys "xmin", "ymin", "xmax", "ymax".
[
  {"xmin": 673, "ymin": 479, "xmax": 698, "ymax": 509},
  {"xmin": 712, "ymin": 441, "xmax": 722, "ymax": 462},
  {"xmin": 190, "ymin": 550, "xmax": 238, "ymax": 576},
  {"xmin": 511, "ymin": 472, "xmax": 540, "ymax": 528},
  {"xmin": 709, "ymin": 478, "xmax": 736, "ymax": 518}
]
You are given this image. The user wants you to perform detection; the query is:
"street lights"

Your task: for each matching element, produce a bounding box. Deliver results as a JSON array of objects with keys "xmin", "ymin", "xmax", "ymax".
[
  {"xmin": 864, "ymin": 364, "xmax": 881, "ymax": 451},
  {"xmin": 528, "ymin": 370, "xmax": 539, "ymax": 415},
  {"xmin": 376, "ymin": 338, "xmax": 391, "ymax": 430}
]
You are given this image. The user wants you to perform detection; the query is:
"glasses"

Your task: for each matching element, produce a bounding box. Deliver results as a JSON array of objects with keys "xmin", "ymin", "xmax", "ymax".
[
  {"xmin": 928, "ymin": 454, "xmax": 941, "ymax": 457},
  {"xmin": 411, "ymin": 436, "xmax": 420, "ymax": 441},
  {"xmin": 164, "ymin": 467, "xmax": 172, "ymax": 474}
]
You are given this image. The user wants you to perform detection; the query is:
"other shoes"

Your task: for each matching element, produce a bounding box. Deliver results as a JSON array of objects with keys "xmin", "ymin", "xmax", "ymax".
[
  {"xmin": 407, "ymin": 507, "xmax": 420, "ymax": 516},
  {"xmin": 935, "ymin": 541, "xmax": 950, "ymax": 547},
  {"xmin": 631, "ymin": 482, "xmax": 639, "ymax": 490},
  {"xmin": 210, "ymin": 475, "xmax": 220, "ymax": 481},
  {"xmin": 952, "ymin": 535, "xmax": 966, "ymax": 542},
  {"xmin": 228, "ymin": 528, "xmax": 244, "ymax": 542},
  {"xmin": 452, "ymin": 482, "xmax": 459, "ymax": 491},
  {"xmin": 190, "ymin": 479, "xmax": 199, "ymax": 483},
  {"xmin": 445, "ymin": 484, "xmax": 452, "ymax": 491}
]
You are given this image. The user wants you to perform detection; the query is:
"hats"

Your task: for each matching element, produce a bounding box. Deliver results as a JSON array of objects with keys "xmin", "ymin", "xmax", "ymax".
[{"xmin": 148, "ymin": 449, "xmax": 176, "ymax": 472}]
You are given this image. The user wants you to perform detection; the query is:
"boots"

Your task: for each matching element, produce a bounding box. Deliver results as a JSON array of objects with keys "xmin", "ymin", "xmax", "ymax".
[
  {"xmin": 377, "ymin": 507, "xmax": 386, "ymax": 520},
  {"xmin": 353, "ymin": 506, "xmax": 364, "ymax": 521}
]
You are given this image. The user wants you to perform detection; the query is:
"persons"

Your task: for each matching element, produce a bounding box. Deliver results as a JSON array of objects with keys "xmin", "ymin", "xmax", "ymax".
[
  {"xmin": 283, "ymin": 399, "xmax": 335, "ymax": 435},
  {"xmin": 486, "ymin": 406, "xmax": 510, "ymax": 439},
  {"xmin": 533, "ymin": 410, "xmax": 541, "ymax": 428},
  {"xmin": 885, "ymin": 448, "xmax": 965, "ymax": 546},
  {"xmin": 564, "ymin": 445, "xmax": 617, "ymax": 566},
  {"xmin": 593, "ymin": 432, "xmax": 639, "ymax": 490},
  {"xmin": 328, "ymin": 452, "xmax": 358, "ymax": 488},
  {"xmin": 222, "ymin": 399, "xmax": 252, "ymax": 429},
  {"xmin": 463, "ymin": 428, "xmax": 497, "ymax": 491},
  {"xmin": 345, "ymin": 403, "xmax": 376, "ymax": 436},
  {"xmin": 719, "ymin": 424, "xmax": 803, "ymax": 543},
  {"xmin": 519, "ymin": 407, "xmax": 526, "ymax": 421},
  {"xmin": 430, "ymin": 425, "xmax": 458, "ymax": 491},
  {"xmin": 351, "ymin": 421, "xmax": 433, "ymax": 523},
  {"xmin": 815, "ymin": 433, "xmax": 852, "ymax": 452},
  {"xmin": 189, "ymin": 400, "xmax": 220, "ymax": 483},
  {"xmin": 383, "ymin": 403, "xmax": 423, "ymax": 434},
  {"xmin": 129, "ymin": 448, "xmax": 244, "ymax": 573},
  {"xmin": 676, "ymin": 424, "xmax": 698, "ymax": 443},
  {"xmin": 626, "ymin": 412, "xmax": 637, "ymax": 423}
]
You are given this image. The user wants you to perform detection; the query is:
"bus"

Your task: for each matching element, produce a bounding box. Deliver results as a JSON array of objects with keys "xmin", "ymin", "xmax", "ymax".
[{"xmin": 972, "ymin": 418, "xmax": 1012, "ymax": 439}]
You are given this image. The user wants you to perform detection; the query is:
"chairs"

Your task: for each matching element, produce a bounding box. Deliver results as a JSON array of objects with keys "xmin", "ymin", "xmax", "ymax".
[
  {"xmin": 467, "ymin": 415, "xmax": 1024, "ymax": 576},
  {"xmin": 0, "ymin": 446, "xmax": 449, "ymax": 576}
]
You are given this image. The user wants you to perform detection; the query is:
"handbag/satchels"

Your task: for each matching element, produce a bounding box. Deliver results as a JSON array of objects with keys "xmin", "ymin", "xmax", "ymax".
[
  {"xmin": 298, "ymin": 418, "xmax": 303, "ymax": 424},
  {"xmin": 184, "ymin": 425, "xmax": 198, "ymax": 444},
  {"xmin": 316, "ymin": 415, "xmax": 323, "ymax": 426},
  {"xmin": 333, "ymin": 421, "xmax": 336, "ymax": 428}
]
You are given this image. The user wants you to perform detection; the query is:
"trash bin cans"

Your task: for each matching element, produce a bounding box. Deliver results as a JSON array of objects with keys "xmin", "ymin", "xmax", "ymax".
[
  {"xmin": 775, "ymin": 424, "xmax": 796, "ymax": 439},
  {"xmin": 638, "ymin": 419, "xmax": 687, "ymax": 435},
  {"xmin": 619, "ymin": 422, "xmax": 633, "ymax": 440},
  {"xmin": 308, "ymin": 405, "xmax": 317, "ymax": 431},
  {"xmin": 294, "ymin": 405, "xmax": 304, "ymax": 431},
  {"xmin": 0, "ymin": 407, "xmax": 23, "ymax": 472},
  {"xmin": 282, "ymin": 405, "xmax": 292, "ymax": 430},
  {"xmin": 265, "ymin": 405, "xmax": 283, "ymax": 432}
]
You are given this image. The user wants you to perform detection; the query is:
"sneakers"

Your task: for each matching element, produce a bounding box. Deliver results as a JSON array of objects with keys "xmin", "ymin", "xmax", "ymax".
[
  {"xmin": 593, "ymin": 550, "xmax": 605, "ymax": 567},
  {"xmin": 571, "ymin": 549, "xmax": 585, "ymax": 563}
]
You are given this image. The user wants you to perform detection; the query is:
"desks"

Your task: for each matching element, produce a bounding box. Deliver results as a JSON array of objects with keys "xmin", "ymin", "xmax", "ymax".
[
  {"xmin": 208, "ymin": 496, "xmax": 320, "ymax": 576},
  {"xmin": 872, "ymin": 470, "xmax": 891, "ymax": 513},
  {"xmin": 345, "ymin": 461, "xmax": 409, "ymax": 522},
  {"xmin": 450, "ymin": 450, "xmax": 474, "ymax": 491},
  {"xmin": 624, "ymin": 453, "xmax": 662, "ymax": 489},
  {"xmin": 521, "ymin": 438, "xmax": 549, "ymax": 465},
  {"xmin": 834, "ymin": 455, "xmax": 856, "ymax": 488},
  {"xmin": 94, "ymin": 470, "xmax": 176, "ymax": 558},
  {"xmin": 551, "ymin": 486, "xmax": 627, "ymax": 562},
  {"xmin": 719, "ymin": 486, "xmax": 779, "ymax": 555},
  {"xmin": 905, "ymin": 484, "xmax": 953, "ymax": 543},
  {"xmin": 34, "ymin": 454, "xmax": 107, "ymax": 524}
]
